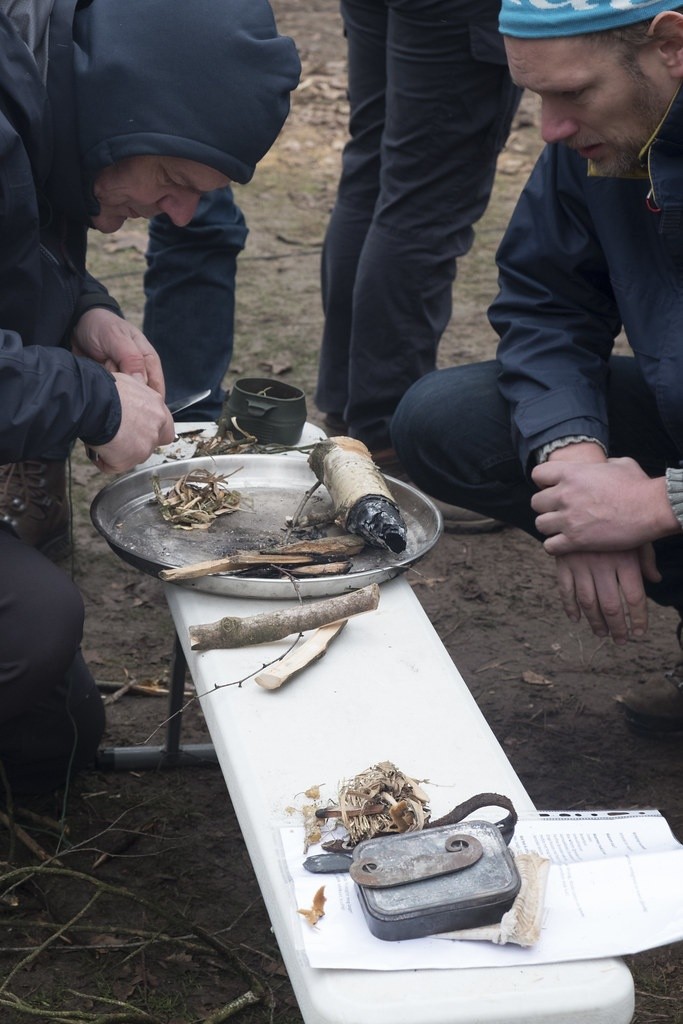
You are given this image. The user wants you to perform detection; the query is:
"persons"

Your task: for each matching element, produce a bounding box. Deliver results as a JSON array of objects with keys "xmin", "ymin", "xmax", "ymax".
[
  {"xmin": 134, "ymin": 165, "xmax": 263, "ymax": 429},
  {"xmin": 312, "ymin": 0, "xmax": 537, "ymax": 532},
  {"xmin": 0, "ymin": 0, "xmax": 306, "ymax": 811},
  {"xmin": 388, "ymin": 0, "xmax": 683, "ymax": 738}
]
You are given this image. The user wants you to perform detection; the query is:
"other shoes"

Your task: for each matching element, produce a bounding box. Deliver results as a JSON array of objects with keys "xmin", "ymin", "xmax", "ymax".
[{"xmin": 622, "ymin": 648, "xmax": 682, "ymax": 739}]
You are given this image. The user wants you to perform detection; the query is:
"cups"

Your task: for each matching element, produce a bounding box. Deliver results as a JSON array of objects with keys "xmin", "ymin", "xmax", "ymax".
[{"xmin": 224, "ymin": 375, "xmax": 310, "ymax": 448}]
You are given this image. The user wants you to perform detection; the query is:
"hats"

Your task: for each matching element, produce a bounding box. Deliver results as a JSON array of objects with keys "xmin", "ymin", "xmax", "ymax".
[{"xmin": 497, "ymin": 0, "xmax": 682, "ymax": 40}]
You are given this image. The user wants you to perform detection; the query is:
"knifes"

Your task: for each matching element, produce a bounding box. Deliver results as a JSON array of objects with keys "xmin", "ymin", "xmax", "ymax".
[{"xmin": 164, "ymin": 390, "xmax": 211, "ymax": 415}]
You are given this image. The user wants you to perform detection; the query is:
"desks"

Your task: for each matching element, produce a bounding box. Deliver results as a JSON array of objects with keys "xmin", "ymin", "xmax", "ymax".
[{"xmin": 92, "ymin": 408, "xmax": 642, "ymax": 1017}]
